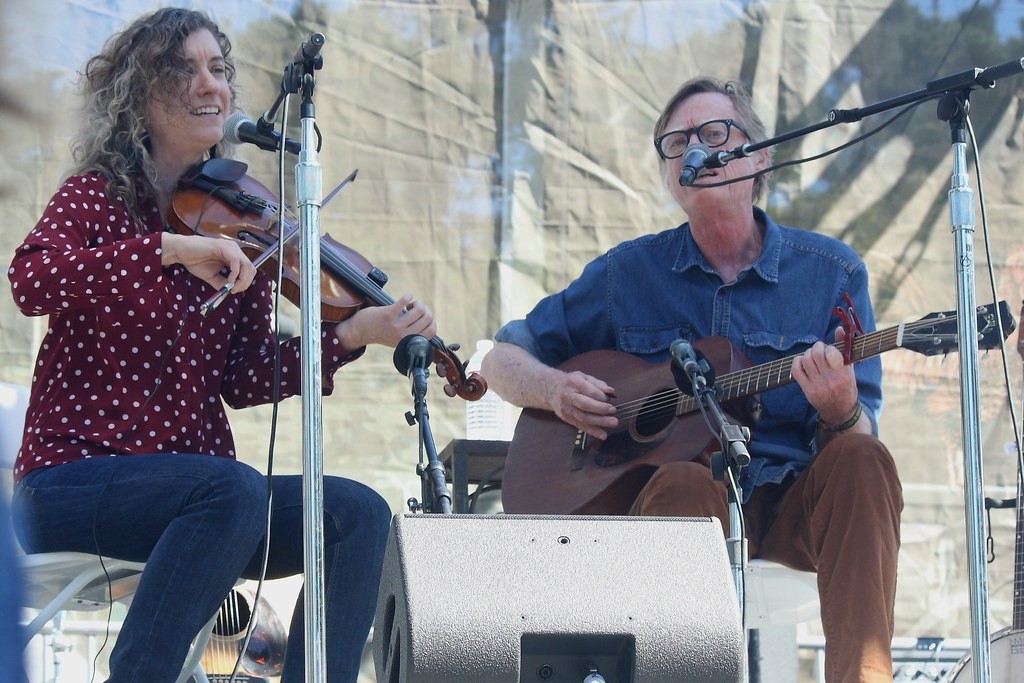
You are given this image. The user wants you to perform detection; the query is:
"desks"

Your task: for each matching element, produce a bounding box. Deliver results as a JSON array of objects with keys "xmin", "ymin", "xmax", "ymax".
[{"xmin": 439, "ymin": 438, "xmax": 509, "ymax": 515}]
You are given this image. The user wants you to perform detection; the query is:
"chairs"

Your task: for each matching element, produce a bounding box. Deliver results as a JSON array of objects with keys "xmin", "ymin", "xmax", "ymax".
[{"xmin": 0, "ymin": 382, "xmax": 217, "ymax": 683}]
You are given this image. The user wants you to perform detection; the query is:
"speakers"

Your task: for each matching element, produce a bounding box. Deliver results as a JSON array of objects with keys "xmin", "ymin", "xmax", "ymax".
[{"xmin": 371, "ymin": 514, "xmax": 747, "ymax": 683}]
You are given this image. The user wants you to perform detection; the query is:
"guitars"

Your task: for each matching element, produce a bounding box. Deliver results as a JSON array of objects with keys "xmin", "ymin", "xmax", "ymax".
[
  {"xmin": 498, "ymin": 299, "xmax": 1016, "ymax": 516},
  {"xmin": 183, "ymin": 586, "xmax": 287, "ymax": 683},
  {"xmin": 945, "ymin": 299, "xmax": 1024, "ymax": 683}
]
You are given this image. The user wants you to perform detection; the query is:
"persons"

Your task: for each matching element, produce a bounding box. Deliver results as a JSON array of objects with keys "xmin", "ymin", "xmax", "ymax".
[
  {"xmin": 8, "ymin": 10, "xmax": 435, "ymax": 683},
  {"xmin": 480, "ymin": 74, "xmax": 904, "ymax": 683}
]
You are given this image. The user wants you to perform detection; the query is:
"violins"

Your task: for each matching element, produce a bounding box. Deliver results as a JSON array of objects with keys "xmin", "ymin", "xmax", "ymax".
[{"xmin": 167, "ymin": 158, "xmax": 489, "ymax": 402}]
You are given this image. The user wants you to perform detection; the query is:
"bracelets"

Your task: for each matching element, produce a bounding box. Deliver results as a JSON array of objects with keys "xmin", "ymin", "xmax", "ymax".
[{"xmin": 815, "ymin": 401, "xmax": 862, "ymax": 432}]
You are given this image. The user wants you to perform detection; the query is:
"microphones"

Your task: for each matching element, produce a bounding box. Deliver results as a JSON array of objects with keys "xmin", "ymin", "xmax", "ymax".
[
  {"xmin": 393, "ymin": 334, "xmax": 433, "ymax": 376},
  {"xmin": 222, "ymin": 111, "xmax": 302, "ymax": 155},
  {"xmin": 678, "ymin": 144, "xmax": 712, "ymax": 187},
  {"xmin": 669, "ymin": 339, "xmax": 715, "ymax": 397}
]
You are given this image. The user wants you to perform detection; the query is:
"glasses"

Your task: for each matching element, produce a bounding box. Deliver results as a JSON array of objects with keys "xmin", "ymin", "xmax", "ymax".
[{"xmin": 653, "ymin": 119, "xmax": 751, "ymax": 162}]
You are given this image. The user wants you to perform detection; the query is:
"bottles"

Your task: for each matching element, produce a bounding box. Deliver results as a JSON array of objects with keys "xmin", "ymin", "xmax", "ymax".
[{"xmin": 464, "ymin": 338, "xmax": 503, "ymax": 441}]
